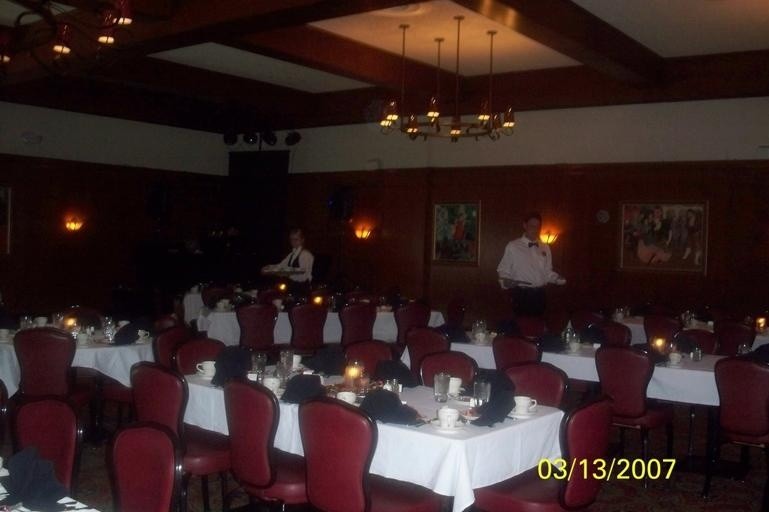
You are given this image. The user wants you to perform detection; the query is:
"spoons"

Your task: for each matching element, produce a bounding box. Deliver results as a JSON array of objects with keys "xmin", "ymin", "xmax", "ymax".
[{"xmin": 56, "ymin": 500, "xmax": 92, "ymax": 511}]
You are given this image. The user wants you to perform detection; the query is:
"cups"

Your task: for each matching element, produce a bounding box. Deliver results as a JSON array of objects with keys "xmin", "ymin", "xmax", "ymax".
[
  {"xmin": 196, "ymin": 360, "xmax": 216, "ymax": 375},
  {"xmin": 563, "ymin": 327, "xmax": 582, "ymax": 353},
  {"xmin": 436, "ymin": 408, "xmax": 461, "ymax": 428},
  {"xmin": 469, "ymin": 319, "xmax": 488, "ymax": 343},
  {"xmin": 336, "ymin": 391, "xmax": 356, "ymax": 405},
  {"xmin": 251, "ymin": 349, "xmax": 293, "ymax": 376},
  {"xmin": 734, "ymin": 343, "xmax": 753, "ymax": 357},
  {"xmin": 433, "ymin": 374, "xmax": 450, "ymax": 404},
  {"xmin": 262, "ymin": 379, "xmax": 282, "ymax": 396},
  {"xmin": 613, "ymin": 305, "xmax": 631, "ymax": 318},
  {"xmin": 212, "ymin": 286, "xmax": 284, "ymax": 314},
  {"xmin": 291, "ymin": 354, "xmax": 302, "ymax": 368},
  {"xmin": 666, "ymin": 352, "xmax": 684, "ymax": 366},
  {"xmin": 118, "ymin": 319, "xmax": 151, "ymax": 344},
  {"xmin": 449, "ymin": 377, "xmax": 464, "ymax": 394},
  {"xmin": 513, "ymin": 396, "xmax": 537, "ymax": 413},
  {"xmin": 0, "ymin": 308, "xmax": 116, "ymax": 348},
  {"xmin": 474, "ymin": 383, "xmax": 491, "ymax": 405}
]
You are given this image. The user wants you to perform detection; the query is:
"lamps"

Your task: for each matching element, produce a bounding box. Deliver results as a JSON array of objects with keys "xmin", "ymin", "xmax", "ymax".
[
  {"xmin": 0, "ymin": 1, "xmax": 135, "ymax": 75},
  {"xmin": 372, "ymin": 12, "xmax": 522, "ymax": 147}
]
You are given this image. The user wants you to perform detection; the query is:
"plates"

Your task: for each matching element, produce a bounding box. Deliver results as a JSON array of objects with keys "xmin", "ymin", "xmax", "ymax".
[
  {"xmin": 508, "ymin": 411, "xmax": 532, "ymax": 421},
  {"xmin": 195, "ymin": 374, "xmax": 213, "ymax": 381},
  {"xmin": 270, "ymin": 388, "xmax": 286, "ymax": 402},
  {"xmin": 428, "ymin": 419, "xmax": 469, "ymax": 434},
  {"xmin": 664, "ymin": 360, "xmax": 686, "ymax": 369}
]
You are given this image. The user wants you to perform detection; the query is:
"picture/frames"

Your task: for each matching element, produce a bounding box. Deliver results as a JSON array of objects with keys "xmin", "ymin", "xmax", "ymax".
[
  {"xmin": 425, "ymin": 197, "xmax": 483, "ymax": 269},
  {"xmin": 615, "ymin": 197, "xmax": 714, "ymax": 277}
]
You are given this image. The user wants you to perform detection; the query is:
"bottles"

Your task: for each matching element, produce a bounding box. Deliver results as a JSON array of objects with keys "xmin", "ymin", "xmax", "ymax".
[
  {"xmin": 691, "ymin": 342, "xmax": 704, "ymax": 362},
  {"xmin": 383, "ymin": 378, "xmax": 399, "ymax": 399},
  {"xmin": 684, "ymin": 311, "xmax": 699, "ymax": 330}
]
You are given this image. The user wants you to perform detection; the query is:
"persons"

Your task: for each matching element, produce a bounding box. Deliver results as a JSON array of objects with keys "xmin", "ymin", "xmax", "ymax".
[
  {"xmin": 451, "ymin": 211, "xmax": 467, "ymax": 242},
  {"xmin": 496, "ymin": 212, "xmax": 566, "ymax": 319},
  {"xmin": 636, "ymin": 206, "xmax": 701, "ymax": 260},
  {"xmin": 259, "ymin": 229, "xmax": 314, "ymax": 286}
]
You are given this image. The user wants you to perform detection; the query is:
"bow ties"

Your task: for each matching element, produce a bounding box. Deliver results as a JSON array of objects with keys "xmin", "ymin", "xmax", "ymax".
[{"xmin": 528, "ymin": 242, "xmax": 538, "ymax": 248}]
